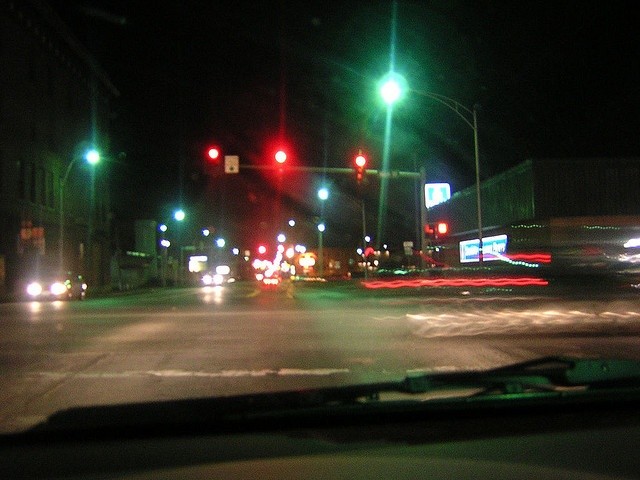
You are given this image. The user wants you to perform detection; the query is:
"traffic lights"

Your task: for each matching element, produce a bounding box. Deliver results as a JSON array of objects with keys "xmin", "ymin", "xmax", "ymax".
[
  {"xmin": 274, "ymin": 150, "xmax": 286, "ymax": 163},
  {"xmin": 354, "ymin": 154, "xmax": 365, "ymax": 180},
  {"xmin": 208, "ymin": 149, "xmax": 218, "ymax": 159}
]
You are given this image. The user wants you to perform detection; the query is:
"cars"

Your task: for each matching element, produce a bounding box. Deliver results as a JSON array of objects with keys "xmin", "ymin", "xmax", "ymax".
[{"xmin": 26, "ymin": 270, "xmax": 86, "ymax": 301}]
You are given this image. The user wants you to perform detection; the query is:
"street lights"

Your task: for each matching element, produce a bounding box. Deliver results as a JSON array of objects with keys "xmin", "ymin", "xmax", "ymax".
[
  {"xmin": 60, "ymin": 148, "xmax": 101, "ymax": 284},
  {"xmin": 318, "ymin": 188, "xmax": 368, "ymax": 280},
  {"xmin": 381, "ymin": 79, "xmax": 483, "ymax": 262}
]
